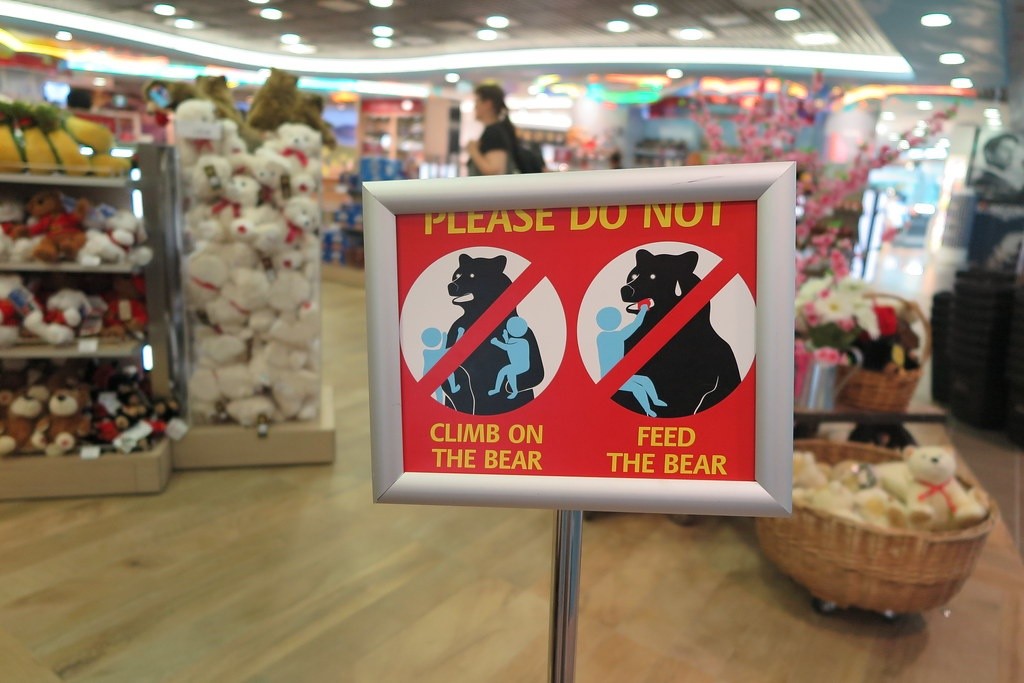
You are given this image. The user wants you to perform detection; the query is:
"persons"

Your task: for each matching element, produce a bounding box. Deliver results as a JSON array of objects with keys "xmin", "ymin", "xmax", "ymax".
[{"xmin": 466, "ymin": 83, "xmax": 517, "ymax": 175}]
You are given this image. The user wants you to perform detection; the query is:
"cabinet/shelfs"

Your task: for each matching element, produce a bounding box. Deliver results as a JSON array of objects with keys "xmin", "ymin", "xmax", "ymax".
[{"xmin": 0, "ymin": 95, "xmax": 690, "ymax": 493}]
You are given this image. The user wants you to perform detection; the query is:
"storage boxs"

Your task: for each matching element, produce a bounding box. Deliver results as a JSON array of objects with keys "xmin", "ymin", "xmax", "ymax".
[{"xmin": 0, "ymin": 52, "xmax": 75, "ymax": 108}]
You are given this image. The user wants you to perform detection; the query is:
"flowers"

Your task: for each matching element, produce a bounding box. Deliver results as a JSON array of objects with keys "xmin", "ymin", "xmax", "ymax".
[{"xmin": 683, "ymin": 66, "xmax": 961, "ymax": 395}]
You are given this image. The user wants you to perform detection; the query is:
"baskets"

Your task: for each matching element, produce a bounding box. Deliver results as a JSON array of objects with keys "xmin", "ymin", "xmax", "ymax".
[
  {"xmin": 840, "ymin": 291, "xmax": 933, "ymax": 416},
  {"xmin": 756, "ymin": 440, "xmax": 998, "ymax": 614}
]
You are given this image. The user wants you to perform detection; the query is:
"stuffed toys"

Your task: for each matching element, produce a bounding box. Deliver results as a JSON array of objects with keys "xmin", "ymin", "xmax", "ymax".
[
  {"xmin": 0, "ymin": 100, "xmax": 189, "ymax": 457},
  {"xmin": 172, "ymin": 97, "xmax": 324, "ymax": 426},
  {"xmin": 791, "ymin": 445, "xmax": 991, "ymax": 529}
]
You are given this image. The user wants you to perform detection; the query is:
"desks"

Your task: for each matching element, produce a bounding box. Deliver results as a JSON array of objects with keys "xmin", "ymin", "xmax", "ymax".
[{"xmin": 794, "ymin": 405, "xmax": 949, "ymax": 440}]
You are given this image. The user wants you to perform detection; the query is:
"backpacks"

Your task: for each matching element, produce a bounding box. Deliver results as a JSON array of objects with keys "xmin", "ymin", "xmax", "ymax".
[{"xmin": 497, "ymin": 115, "xmax": 545, "ymax": 172}]
